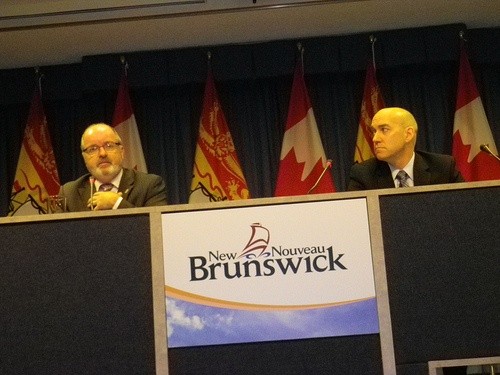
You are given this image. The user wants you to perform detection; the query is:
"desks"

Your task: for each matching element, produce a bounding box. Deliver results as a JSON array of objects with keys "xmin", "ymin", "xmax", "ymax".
[{"xmin": 0, "ymin": 179, "xmax": 500, "ymax": 375}]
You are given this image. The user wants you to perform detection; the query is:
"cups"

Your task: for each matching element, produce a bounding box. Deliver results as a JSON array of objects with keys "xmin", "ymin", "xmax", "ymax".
[{"xmin": 47, "ymin": 195, "xmax": 67, "ymax": 215}]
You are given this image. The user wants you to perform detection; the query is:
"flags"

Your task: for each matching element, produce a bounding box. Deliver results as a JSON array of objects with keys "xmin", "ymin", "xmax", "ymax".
[
  {"xmin": 188, "ymin": 65, "xmax": 252, "ymax": 205},
  {"xmin": 452, "ymin": 50, "xmax": 500, "ymax": 182},
  {"xmin": 9, "ymin": 82, "xmax": 64, "ymax": 217},
  {"xmin": 274, "ymin": 61, "xmax": 335, "ymax": 197},
  {"xmin": 111, "ymin": 74, "xmax": 147, "ymax": 174},
  {"xmin": 355, "ymin": 63, "xmax": 387, "ymax": 163}
]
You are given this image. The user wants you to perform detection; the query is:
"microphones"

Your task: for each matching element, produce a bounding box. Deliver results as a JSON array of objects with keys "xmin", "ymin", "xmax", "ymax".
[
  {"xmin": 480, "ymin": 144, "xmax": 500, "ymax": 161},
  {"xmin": 89, "ymin": 176, "xmax": 95, "ymax": 210},
  {"xmin": 307, "ymin": 159, "xmax": 333, "ymax": 194}
]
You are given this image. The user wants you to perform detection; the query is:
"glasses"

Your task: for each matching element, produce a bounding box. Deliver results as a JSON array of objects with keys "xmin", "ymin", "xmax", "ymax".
[{"xmin": 82, "ymin": 141, "xmax": 122, "ymax": 155}]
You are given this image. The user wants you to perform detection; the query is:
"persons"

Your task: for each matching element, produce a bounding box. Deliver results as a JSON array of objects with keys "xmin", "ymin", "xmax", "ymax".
[
  {"xmin": 346, "ymin": 108, "xmax": 465, "ymax": 192},
  {"xmin": 53, "ymin": 124, "xmax": 166, "ymax": 214}
]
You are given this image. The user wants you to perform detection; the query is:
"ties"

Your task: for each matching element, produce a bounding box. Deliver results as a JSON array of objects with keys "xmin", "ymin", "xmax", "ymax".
[
  {"xmin": 396, "ymin": 170, "xmax": 409, "ymax": 188},
  {"xmin": 100, "ymin": 184, "xmax": 113, "ymax": 192}
]
